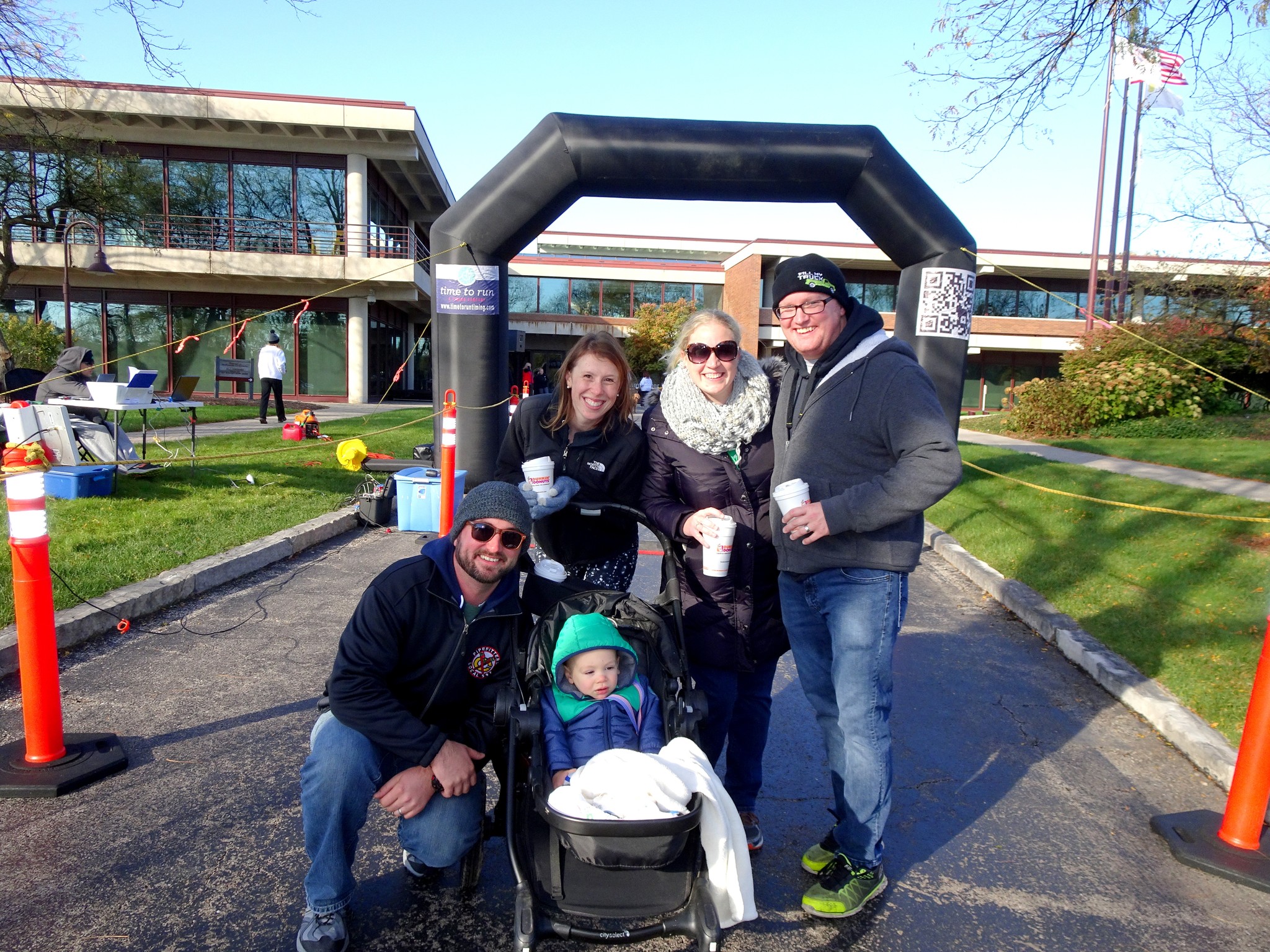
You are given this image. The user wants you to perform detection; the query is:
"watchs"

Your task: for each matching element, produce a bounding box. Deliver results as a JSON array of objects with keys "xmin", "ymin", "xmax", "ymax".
[{"xmin": 431, "ymin": 774, "xmax": 441, "ymax": 792}]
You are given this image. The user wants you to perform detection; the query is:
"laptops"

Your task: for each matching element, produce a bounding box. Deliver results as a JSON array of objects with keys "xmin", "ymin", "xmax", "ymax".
[
  {"xmin": 153, "ymin": 376, "xmax": 200, "ymax": 401},
  {"xmin": 58, "ymin": 374, "xmax": 116, "ymax": 400}
]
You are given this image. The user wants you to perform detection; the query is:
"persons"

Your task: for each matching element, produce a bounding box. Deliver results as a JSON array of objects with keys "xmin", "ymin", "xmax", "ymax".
[
  {"xmin": 296, "ymin": 481, "xmax": 532, "ymax": 952},
  {"xmin": 540, "ymin": 612, "xmax": 660, "ymax": 789},
  {"xmin": 641, "ymin": 310, "xmax": 792, "ymax": 852},
  {"xmin": 640, "ymin": 371, "xmax": 652, "ymax": 408},
  {"xmin": 770, "ymin": 253, "xmax": 961, "ymax": 916},
  {"xmin": 258, "ymin": 329, "xmax": 286, "ymax": 424},
  {"xmin": 509, "ymin": 362, "xmax": 548, "ymax": 397},
  {"xmin": 493, "ymin": 330, "xmax": 647, "ymax": 628},
  {"xmin": 35, "ymin": 346, "xmax": 165, "ymax": 479}
]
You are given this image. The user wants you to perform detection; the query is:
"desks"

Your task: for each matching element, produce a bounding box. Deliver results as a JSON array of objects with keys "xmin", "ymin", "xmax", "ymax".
[{"xmin": 46, "ymin": 400, "xmax": 204, "ymax": 493}]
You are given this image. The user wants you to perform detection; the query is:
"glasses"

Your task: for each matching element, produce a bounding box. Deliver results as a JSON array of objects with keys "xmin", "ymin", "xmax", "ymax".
[
  {"xmin": 83, "ymin": 362, "xmax": 95, "ymax": 366},
  {"xmin": 465, "ymin": 521, "xmax": 528, "ymax": 552},
  {"xmin": 774, "ymin": 292, "xmax": 832, "ymax": 319},
  {"xmin": 687, "ymin": 339, "xmax": 743, "ymax": 363}
]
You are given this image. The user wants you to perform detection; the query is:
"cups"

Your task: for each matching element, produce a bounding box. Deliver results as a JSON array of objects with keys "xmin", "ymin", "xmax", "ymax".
[
  {"xmin": 521, "ymin": 456, "xmax": 554, "ymax": 506},
  {"xmin": 533, "ymin": 559, "xmax": 567, "ymax": 583},
  {"xmin": 773, "ymin": 477, "xmax": 811, "ymax": 534},
  {"xmin": 703, "ymin": 515, "xmax": 737, "ymax": 577}
]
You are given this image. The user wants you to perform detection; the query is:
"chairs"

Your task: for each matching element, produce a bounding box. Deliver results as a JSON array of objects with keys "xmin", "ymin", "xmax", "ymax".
[{"xmin": 2, "ymin": 367, "xmax": 108, "ymax": 462}]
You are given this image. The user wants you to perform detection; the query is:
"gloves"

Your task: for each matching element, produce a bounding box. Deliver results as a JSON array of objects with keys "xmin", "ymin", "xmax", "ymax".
[
  {"xmin": 92, "ymin": 416, "xmax": 107, "ymax": 426},
  {"xmin": 530, "ymin": 475, "xmax": 579, "ymax": 521},
  {"xmin": 516, "ymin": 481, "xmax": 539, "ymax": 512}
]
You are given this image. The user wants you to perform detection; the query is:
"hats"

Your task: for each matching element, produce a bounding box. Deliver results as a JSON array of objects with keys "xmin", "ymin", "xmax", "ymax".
[
  {"xmin": 82, "ymin": 351, "xmax": 94, "ymax": 362},
  {"xmin": 269, "ymin": 330, "xmax": 279, "ymax": 344},
  {"xmin": 772, "ymin": 253, "xmax": 850, "ymax": 323},
  {"xmin": 451, "ymin": 480, "xmax": 532, "ymax": 556}
]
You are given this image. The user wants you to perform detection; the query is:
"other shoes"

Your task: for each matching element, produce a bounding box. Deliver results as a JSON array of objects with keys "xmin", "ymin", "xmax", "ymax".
[
  {"xmin": 278, "ymin": 416, "xmax": 287, "ymax": 423},
  {"xmin": 260, "ymin": 416, "xmax": 268, "ymax": 424}
]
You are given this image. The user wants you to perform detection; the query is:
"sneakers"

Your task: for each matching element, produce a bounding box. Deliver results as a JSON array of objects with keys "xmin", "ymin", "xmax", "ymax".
[
  {"xmin": 127, "ymin": 462, "xmax": 164, "ymax": 474},
  {"xmin": 738, "ymin": 808, "xmax": 763, "ymax": 850},
  {"xmin": 296, "ymin": 903, "xmax": 354, "ymax": 952},
  {"xmin": 802, "ymin": 851, "xmax": 888, "ymax": 918},
  {"xmin": 403, "ymin": 849, "xmax": 426, "ymax": 876},
  {"xmin": 801, "ymin": 807, "xmax": 844, "ymax": 875}
]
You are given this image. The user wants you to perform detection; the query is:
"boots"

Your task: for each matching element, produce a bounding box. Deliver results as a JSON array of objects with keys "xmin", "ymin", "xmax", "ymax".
[{"xmin": 492, "ymin": 757, "xmax": 535, "ymax": 821}]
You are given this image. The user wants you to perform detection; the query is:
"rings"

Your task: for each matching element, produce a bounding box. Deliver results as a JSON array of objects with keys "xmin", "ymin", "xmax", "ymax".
[
  {"xmin": 695, "ymin": 523, "xmax": 703, "ymax": 531},
  {"xmin": 398, "ymin": 808, "xmax": 404, "ymax": 816},
  {"xmin": 804, "ymin": 525, "xmax": 810, "ymax": 533}
]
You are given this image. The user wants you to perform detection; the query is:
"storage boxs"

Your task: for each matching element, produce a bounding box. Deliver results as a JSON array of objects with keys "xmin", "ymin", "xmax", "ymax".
[
  {"xmin": 394, "ymin": 465, "xmax": 470, "ymax": 535},
  {"xmin": 45, "ymin": 462, "xmax": 118, "ymax": 501},
  {"xmin": 356, "ymin": 474, "xmax": 395, "ymax": 526}
]
